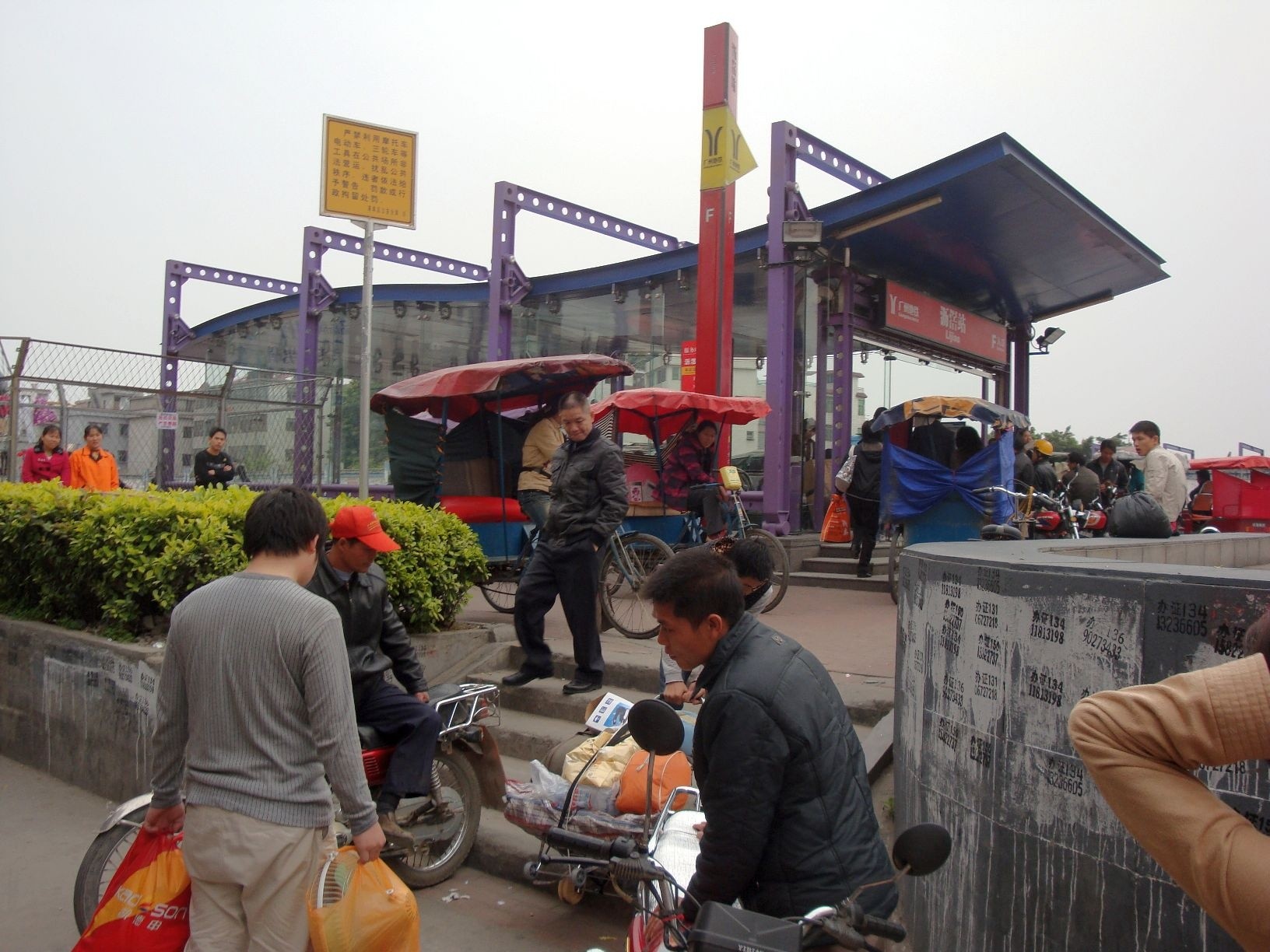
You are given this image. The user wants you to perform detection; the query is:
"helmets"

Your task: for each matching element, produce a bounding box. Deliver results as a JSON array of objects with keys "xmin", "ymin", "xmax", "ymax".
[{"xmin": 1033, "ymin": 440, "xmax": 1053, "ymax": 456}]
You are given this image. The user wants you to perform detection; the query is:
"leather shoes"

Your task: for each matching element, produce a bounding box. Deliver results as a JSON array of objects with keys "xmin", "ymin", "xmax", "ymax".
[
  {"xmin": 503, "ymin": 668, "xmax": 554, "ymax": 685},
  {"xmin": 563, "ymin": 678, "xmax": 602, "ymax": 695}
]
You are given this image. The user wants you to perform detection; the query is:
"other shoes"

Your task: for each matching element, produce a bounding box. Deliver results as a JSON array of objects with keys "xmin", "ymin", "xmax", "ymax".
[
  {"xmin": 600, "ymin": 615, "xmax": 622, "ymax": 632},
  {"xmin": 850, "ymin": 547, "xmax": 860, "ymax": 560},
  {"xmin": 858, "ymin": 572, "xmax": 872, "ymax": 577},
  {"xmin": 706, "ymin": 534, "xmax": 732, "ymax": 549}
]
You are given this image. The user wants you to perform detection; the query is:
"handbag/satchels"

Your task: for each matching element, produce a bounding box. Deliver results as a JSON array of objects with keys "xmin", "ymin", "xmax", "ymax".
[
  {"xmin": 502, "ymin": 717, "xmax": 694, "ymax": 857},
  {"xmin": 835, "ymin": 444, "xmax": 856, "ymax": 492},
  {"xmin": 306, "ymin": 845, "xmax": 421, "ymax": 952},
  {"xmin": 821, "ymin": 493, "xmax": 852, "ymax": 544},
  {"xmin": 1107, "ymin": 490, "xmax": 1171, "ymax": 540},
  {"xmin": 69, "ymin": 822, "xmax": 192, "ymax": 952}
]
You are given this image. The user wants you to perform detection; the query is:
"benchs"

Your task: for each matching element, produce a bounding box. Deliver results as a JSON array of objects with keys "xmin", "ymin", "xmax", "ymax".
[{"xmin": 436, "ymin": 457, "xmax": 529, "ymax": 525}]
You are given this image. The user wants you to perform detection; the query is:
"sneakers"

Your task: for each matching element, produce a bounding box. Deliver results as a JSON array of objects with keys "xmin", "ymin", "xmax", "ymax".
[{"xmin": 379, "ymin": 813, "xmax": 414, "ymax": 850}]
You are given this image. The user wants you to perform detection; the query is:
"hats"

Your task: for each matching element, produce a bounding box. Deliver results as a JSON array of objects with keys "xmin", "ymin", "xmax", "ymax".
[{"xmin": 331, "ymin": 506, "xmax": 401, "ymax": 553}]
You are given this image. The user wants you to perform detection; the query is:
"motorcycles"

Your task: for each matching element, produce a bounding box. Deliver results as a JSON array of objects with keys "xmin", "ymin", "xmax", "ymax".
[
  {"xmin": 73, "ymin": 679, "xmax": 507, "ymax": 939},
  {"xmin": 523, "ymin": 687, "xmax": 954, "ymax": 952}
]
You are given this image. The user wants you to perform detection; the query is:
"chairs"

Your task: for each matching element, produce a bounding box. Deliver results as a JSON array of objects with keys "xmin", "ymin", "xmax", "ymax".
[{"xmin": 1182, "ymin": 481, "xmax": 1213, "ymax": 512}]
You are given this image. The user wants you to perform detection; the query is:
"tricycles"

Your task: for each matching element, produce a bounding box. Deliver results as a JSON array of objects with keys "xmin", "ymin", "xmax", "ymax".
[
  {"xmin": 870, "ymin": 394, "xmax": 1270, "ymax": 604},
  {"xmin": 588, "ymin": 387, "xmax": 791, "ymax": 614},
  {"xmin": 368, "ymin": 351, "xmax": 677, "ymax": 640}
]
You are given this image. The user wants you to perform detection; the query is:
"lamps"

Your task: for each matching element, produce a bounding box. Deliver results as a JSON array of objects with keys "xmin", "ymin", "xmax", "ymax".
[
  {"xmin": 676, "ymin": 270, "xmax": 690, "ymax": 293},
  {"xmin": 269, "ymin": 314, "xmax": 283, "ymax": 330},
  {"xmin": 392, "ymin": 301, "xmax": 407, "ymax": 319},
  {"xmin": 1029, "ymin": 327, "xmax": 1065, "ymax": 355},
  {"xmin": 436, "ymin": 301, "xmax": 452, "ymax": 320},
  {"xmin": 545, "ymin": 294, "xmax": 562, "ymax": 314},
  {"xmin": 237, "ymin": 323, "xmax": 249, "ymax": 339},
  {"xmin": 347, "ymin": 305, "xmax": 361, "ymax": 320},
  {"xmin": 611, "ymin": 282, "xmax": 626, "ymax": 304},
  {"xmin": 756, "ymin": 357, "xmax": 764, "ymax": 370},
  {"xmin": 662, "ymin": 353, "xmax": 670, "ymax": 366}
]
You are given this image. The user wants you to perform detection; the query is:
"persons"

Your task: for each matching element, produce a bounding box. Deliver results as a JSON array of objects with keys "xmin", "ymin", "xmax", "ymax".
[
  {"xmin": 301, "ymin": 505, "xmax": 444, "ymax": 827},
  {"xmin": 653, "ymin": 539, "xmax": 773, "ymax": 711},
  {"xmin": 501, "ymin": 390, "xmax": 630, "ymax": 694},
  {"xmin": 193, "ymin": 427, "xmax": 235, "ymax": 491},
  {"xmin": 801, "ymin": 407, "xmax": 1212, "ymax": 577},
  {"xmin": 20, "ymin": 425, "xmax": 134, "ymax": 492},
  {"xmin": 149, "ymin": 483, "xmax": 388, "ymax": 952},
  {"xmin": 653, "ymin": 419, "xmax": 727, "ymax": 544},
  {"xmin": 650, "ymin": 550, "xmax": 899, "ymax": 951},
  {"xmin": 1069, "ymin": 611, "xmax": 1270, "ymax": 952}
]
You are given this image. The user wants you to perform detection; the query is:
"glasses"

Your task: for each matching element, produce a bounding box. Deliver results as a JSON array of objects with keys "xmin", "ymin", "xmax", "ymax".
[{"xmin": 741, "ymin": 583, "xmax": 762, "ymax": 592}]
